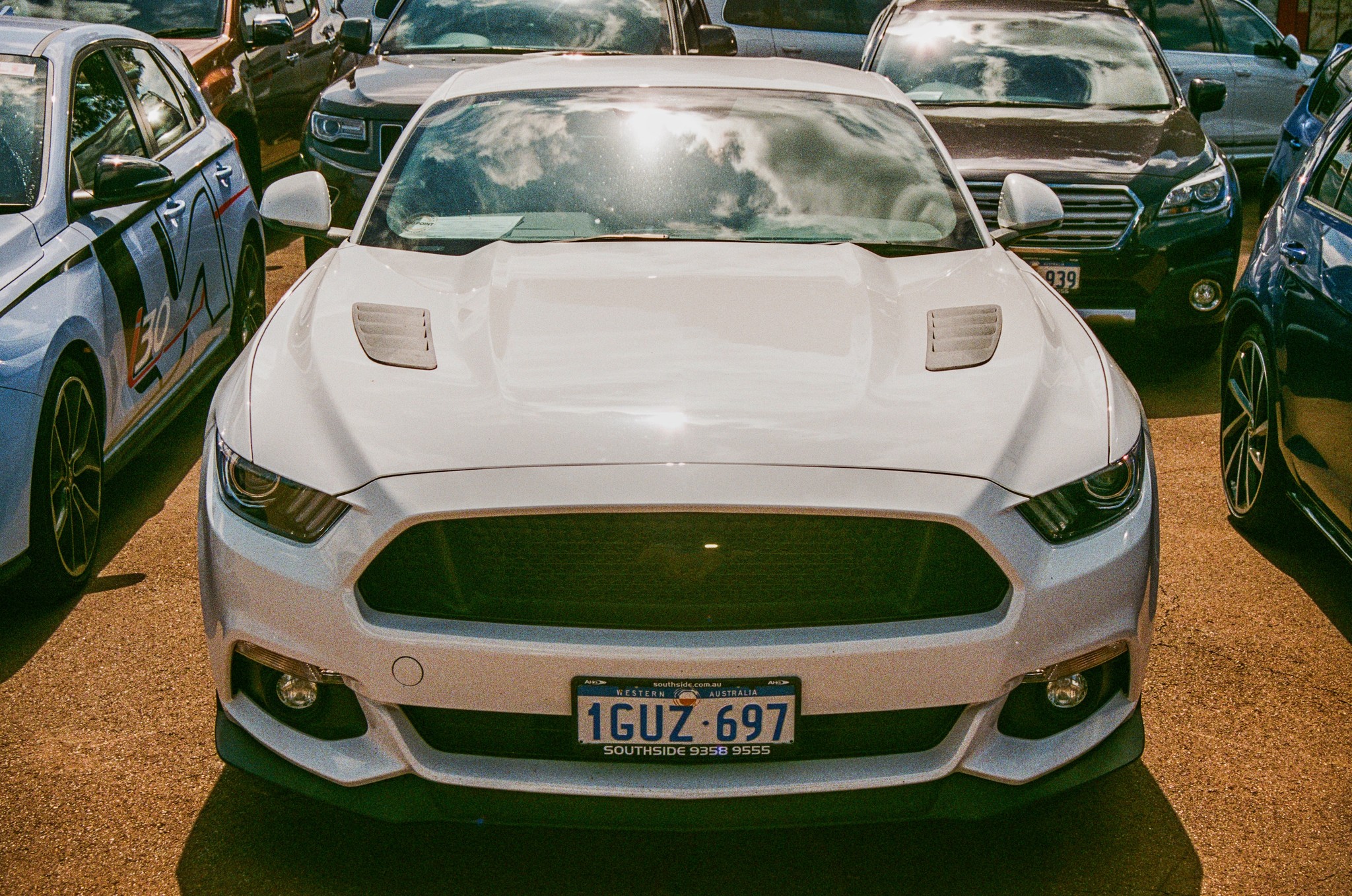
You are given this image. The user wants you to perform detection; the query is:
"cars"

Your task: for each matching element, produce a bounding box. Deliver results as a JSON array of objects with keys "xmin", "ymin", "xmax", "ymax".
[
  {"xmin": 0, "ymin": 13, "xmax": 270, "ymax": 597},
  {"xmin": 197, "ymin": 52, "xmax": 1161, "ymax": 835},
  {"xmin": 6, "ymin": 0, "xmax": 358, "ymax": 210},
  {"xmin": 1131, "ymin": 0, "xmax": 1320, "ymax": 170},
  {"xmin": 707, "ymin": 0, "xmax": 892, "ymax": 69},
  {"xmin": 1220, "ymin": 95, "xmax": 1351, "ymax": 562}
]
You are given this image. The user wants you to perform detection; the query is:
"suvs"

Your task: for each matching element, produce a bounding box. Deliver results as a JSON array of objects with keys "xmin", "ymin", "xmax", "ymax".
[
  {"xmin": 295, "ymin": 0, "xmax": 741, "ymax": 274},
  {"xmin": 862, "ymin": 0, "xmax": 1245, "ymax": 354}
]
